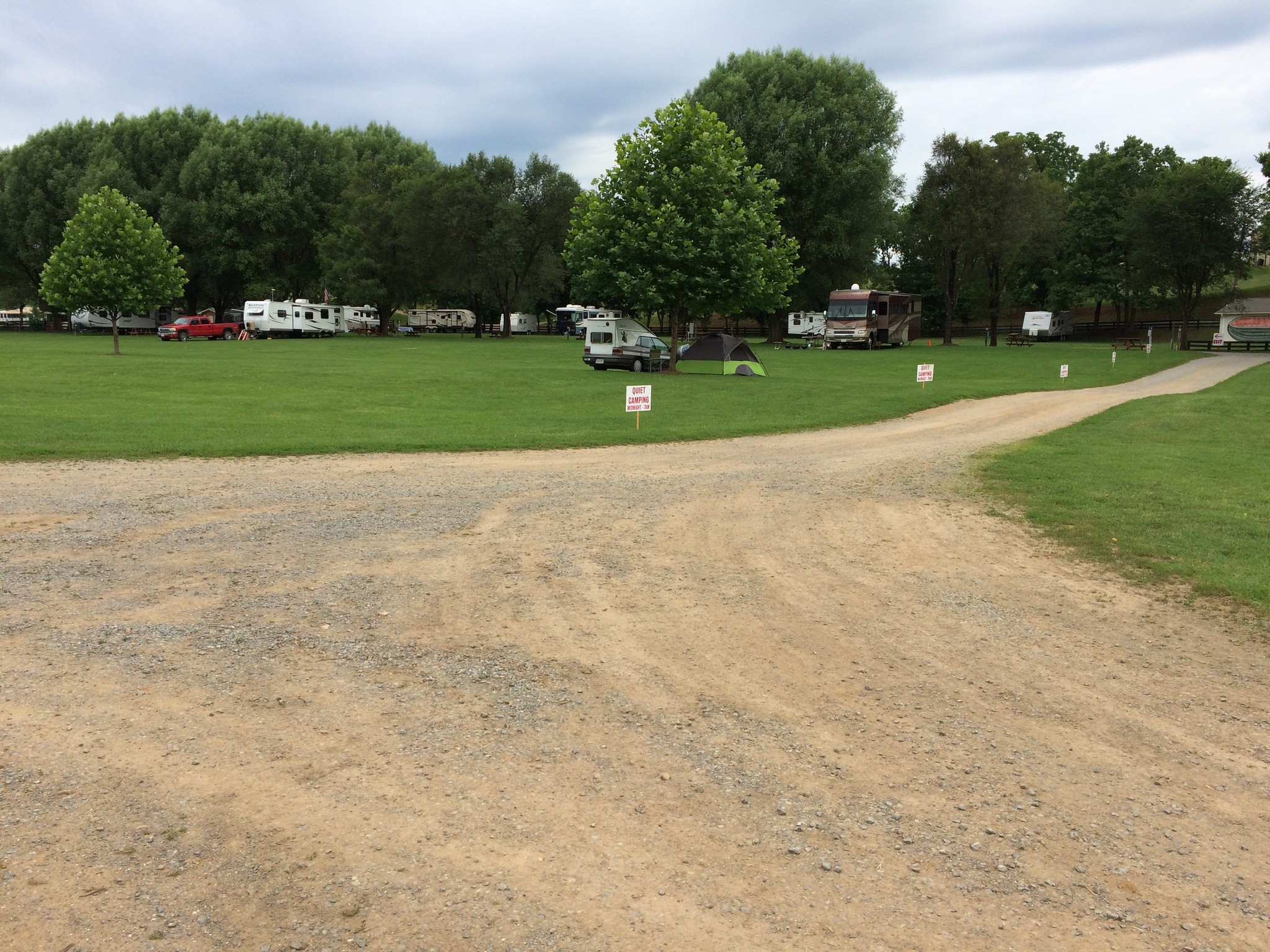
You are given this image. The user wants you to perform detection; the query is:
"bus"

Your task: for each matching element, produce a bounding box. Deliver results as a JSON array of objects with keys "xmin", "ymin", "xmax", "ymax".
[{"xmin": 555, "ymin": 304, "xmax": 622, "ymax": 336}]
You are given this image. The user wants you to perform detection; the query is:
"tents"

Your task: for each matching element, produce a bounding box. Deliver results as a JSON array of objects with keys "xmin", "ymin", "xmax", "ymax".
[{"xmin": 675, "ymin": 333, "xmax": 770, "ymax": 377}]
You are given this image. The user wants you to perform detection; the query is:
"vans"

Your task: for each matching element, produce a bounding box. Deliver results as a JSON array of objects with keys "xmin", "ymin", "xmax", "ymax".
[{"xmin": 582, "ymin": 317, "xmax": 680, "ymax": 373}]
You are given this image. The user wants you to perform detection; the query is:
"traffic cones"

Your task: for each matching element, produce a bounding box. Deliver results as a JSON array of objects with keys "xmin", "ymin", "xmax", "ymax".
[{"xmin": 927, "ymin": 339, "xmax": 932, "ymax": 347}]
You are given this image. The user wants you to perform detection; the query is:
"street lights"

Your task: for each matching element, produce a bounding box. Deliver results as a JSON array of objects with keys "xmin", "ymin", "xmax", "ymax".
[{"xmin": 271, "ymin": 288, "xmax": 276, "ymax": 301}]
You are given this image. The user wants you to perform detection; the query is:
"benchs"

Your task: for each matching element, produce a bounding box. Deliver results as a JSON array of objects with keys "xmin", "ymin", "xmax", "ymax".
[
  {"xmin": 677, "ymin": 339, "xmax": 688, "ymax": 341},
  {"xmin": 1110, "ymin": 344, "xmax": 1146, "ymax": 348},
  {"xmin": 575, "ymin": 338, "xmax": 579, "ymax": 340},
  {"xmin": 1005, "ymin": 343, "xmax": 1035, "ymax": 346},
  {"xmin": 1018, "ymin": 336, "xmax": 1024, "ymax": 338},
  {"xmin": 487, "ymin": 335, "xmax": 500, "ymax": 337},
  {"xmin": 353, "ymin": 331, "xmax": 381, "ymax": 335},
  {"xmin": 405, "ymin": 335, "xmax": 421, "ymax": 337},
  {"xmin": 127, "ymin": 332, "xmax": 155, "ymax": 334},
  {"xmin": 1004, "ymin": 336, "xmax": 1011, "ymax": 339}
]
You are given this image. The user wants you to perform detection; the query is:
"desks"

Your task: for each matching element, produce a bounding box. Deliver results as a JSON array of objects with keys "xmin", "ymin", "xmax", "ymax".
[
  {"xmin": 678, "ymin": 334, "xmax": 686, "ymax": 342},
  {"xmin": 773, "ymin": 342, "xmax": 783, "ymax": 349},
  {"xmin": 357, "ymin": 328, "xmax": 379, "ymax": 337},
  {"xmin": 129, "ymin": 327, "xmax": 152, "ymax": 336},
  {"xmin": 1115, "ymin": 338, "xmax": 1143, "ymax": 351},
  {"xmin": 579, "ymin": 334, "xmax": 586, "ymax": 340},
  {"xmin": 398, "ymin": 327, "xmax": 413, "ymax": 333},
  {"xmin": 801, "ymin": 336, "xmax": 819, "ymax": 346},
  {"xmin": 406, "ymin": 331, "xmax": 419, "ymax": 337},
  {"xmin": 1009, "ymin": 338, "xmax": 1030, "ymax": 347},
  {"xmin": 1007, "ymin": 333, "xmax": 1021, "ymax": 341},
  {"xmin": 490, "ymin": 331, "xmax": 503, "ymax": 339}
]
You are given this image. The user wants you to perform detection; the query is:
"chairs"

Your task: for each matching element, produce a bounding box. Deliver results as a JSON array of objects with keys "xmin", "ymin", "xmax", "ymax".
[{"xmin": 648, "ymin": 349, "xmax": 662, "ymax": 373}]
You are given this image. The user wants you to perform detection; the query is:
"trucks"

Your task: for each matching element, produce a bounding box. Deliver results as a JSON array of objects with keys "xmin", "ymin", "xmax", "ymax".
[
  {"xmin": 823, "ymin": 283, "xmax": 923, "ymax": 351},
  {"xmin": 408, "ymin": 309, "xmax": 485, "ymax": 333}
]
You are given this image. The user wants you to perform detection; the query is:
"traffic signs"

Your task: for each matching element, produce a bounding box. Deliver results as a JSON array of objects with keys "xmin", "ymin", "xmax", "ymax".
[{"xmin": 1212, "ymin": 333, "xmax": 1224, "ymax": 346}]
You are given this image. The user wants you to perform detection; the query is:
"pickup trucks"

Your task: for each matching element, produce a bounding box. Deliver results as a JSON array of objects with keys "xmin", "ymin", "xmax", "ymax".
[{"xmin": 157, "ymin": 316, "xmax": 239, "ymax": 342}]
